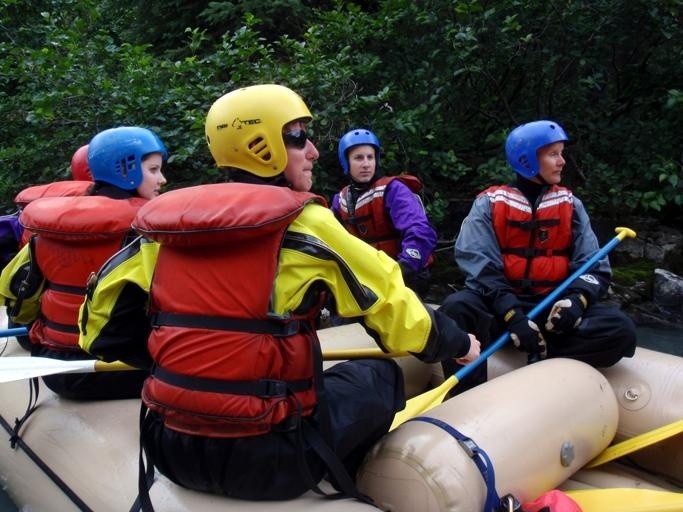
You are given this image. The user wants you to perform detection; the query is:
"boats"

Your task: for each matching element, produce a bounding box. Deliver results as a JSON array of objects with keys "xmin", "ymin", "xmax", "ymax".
[{"xmin": 2, "ymin": 304, "xmax": 683, "ymax": 510}]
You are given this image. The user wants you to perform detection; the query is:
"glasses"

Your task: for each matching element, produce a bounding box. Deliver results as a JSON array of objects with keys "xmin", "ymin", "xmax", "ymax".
[{"xmin": 283, "ymin": 129, "xmax": 309, "ymax": 148}]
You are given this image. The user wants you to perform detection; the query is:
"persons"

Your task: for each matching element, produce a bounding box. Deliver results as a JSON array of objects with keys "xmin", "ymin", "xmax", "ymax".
[
  {"xmin": 1, "ymin": 145, "xmax": 98, "ymax": 354},
  {"xmin": 129, "ymin": 82, "xmax": 480, "ymax": 499},
  {"xmin": 434, "ymin": 119, "xmax": 638, "ymax": 396},
  {"xmin": 20, "ymin": 127, "xmax": 169, "ymax": 397},
  {"xmin": 331, "ymin": 129, "xmax": 435, "ymax": 304}
]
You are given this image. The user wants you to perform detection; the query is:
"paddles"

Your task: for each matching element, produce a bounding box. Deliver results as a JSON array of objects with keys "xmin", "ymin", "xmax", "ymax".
[
  {"xmin": 388, "ymin": 226, "xmax": 637, "ymax": 433},
  {"xmin": 0, "ymin": 347, "xmax": 413, "ymax": 384}
]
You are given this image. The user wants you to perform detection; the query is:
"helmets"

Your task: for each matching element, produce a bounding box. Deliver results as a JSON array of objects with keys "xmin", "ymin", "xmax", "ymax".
[
  {"xmin": 88, "ymin": 127, "xmax": 165, "ymax": 189},
  {"xmin": 339, "ymin": 129, "xmax": 382, "ymax": 174},
  {"xmin": 71, "ymin": 144, "xmax": 93, "ymax": 182},
  {"xmin": 505, "ymin": 120, "xmax": 567, "ymax": 177},
  {"xmin": 206, "ymin": 85, "xmax": 313, "ymax": 178}
]
